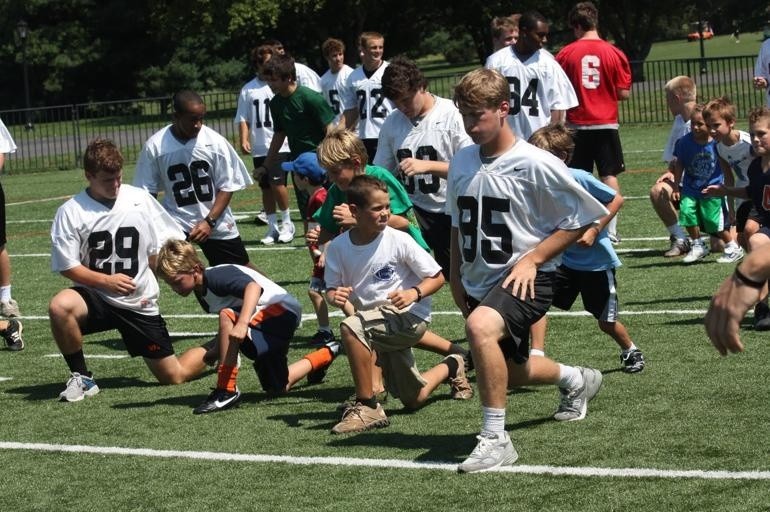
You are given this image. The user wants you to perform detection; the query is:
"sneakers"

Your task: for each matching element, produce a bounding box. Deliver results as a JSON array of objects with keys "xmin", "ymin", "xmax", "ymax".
[
  {"xmin": 58, "ymin": 371, "xmax": 100, "ymax": 401},
  {"xmin": 608, "ymin": 233, "xmax": 621, "ymax": 245},
  {"xmin": 754, "ymin": 304, "xmax": 770, "ymax": 331},
  {"xmin": 458, "ymin": 430, "xmax": 518, "ymax": 473},
  {"xmin": 192, "ymin": 386, "xmax": 241, "ymax": 414},
  {"xmin": 331, "ymin": 389, "xmax": 389, "ymax": 433},
  {"xmin": 621, "ymin": 348, "xmax": 645, "ymax": 374},
  {"xmin": 663, "ymin": 236, "xmax": 744, "ymax": 263},
  {"xmin": 0, "ymin": 299, "xmax": 25, "ymax": 351},
  {"xmin": 553, "ymin": 364, "xmax": 603, "ymax": 421},
  {"xmin": 308, "ymin": 329, "xmax": 344, "ymax": 383},
  {"xmin": 254, "ymin": 212, "xmax": 295, "ymax": 245},
  {"xmin": 445, "ymin": 354, "xmax": 474, "ymax": 400}
]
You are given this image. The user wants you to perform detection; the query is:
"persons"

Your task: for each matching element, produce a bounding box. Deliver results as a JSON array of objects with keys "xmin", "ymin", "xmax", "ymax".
[
  {"xmin": 131, "ymin": 90, "xmax": 271, "ymax": 284},
  {"xmin": 737, "ymin": 106, "xmax": 769, "ymax": 331},
  {"xmin": 1, "ymin": 119, "xmax": 21, "ymax": 319},
  {"xmin": 155, "ymin": 235, "xmax": 345, "ymax": 414},
  {"xmin": 235, "ymin": 33, "xmax": 399, "ymax": 242},
  {"xmin": 317, "ymin": 129, "xmax": 474, "ymax": 373},
  {"xmin": 706, "ymin": 242, "xmax": 770, "ymax": 356},
  {"xmin": 373, "ymin": 61, "xmax": 473, "ymax": 278},
  {"xmin": 318, "ymin": 176, "xmax": 476, "ymax": 435},
  {"xmin": 45, "ymin": 137, "xmax": 242, "ymax": 402},
  {"xmin": 1, "ymin": 315, "xmax": 28, "ymax": 353},
  {"xmin": 753, "ymin": 40, "xmax": 769, "ymax": 87},
  {"xmin": 280, "ymin": 154, "xmax": 334, "ymax": 345},
  {"xmin": 528, "ymin": 123, "xmax": 645, "ymax": 372},
  {"xmin": 650, "ymin": 75, "xmax": 755, "ymax": 263},
  {"xmin": 486, "ymin": 0, "xmax": 632, "ymax": 245},
  {"xmin": 443, "ymin": 67, "xmax": 608, "ymax": 475}
]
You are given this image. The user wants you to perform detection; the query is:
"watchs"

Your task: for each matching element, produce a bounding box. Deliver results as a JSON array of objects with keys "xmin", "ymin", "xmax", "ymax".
[{"xmin": 204, "ymin": 216, "xmax": 216, "ymax": 229}]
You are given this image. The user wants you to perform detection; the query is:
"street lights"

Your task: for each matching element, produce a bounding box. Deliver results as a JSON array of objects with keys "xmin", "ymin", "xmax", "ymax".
[{"xmin": 16, "ymin": 17, "xmax": 33, "ymax": 129}]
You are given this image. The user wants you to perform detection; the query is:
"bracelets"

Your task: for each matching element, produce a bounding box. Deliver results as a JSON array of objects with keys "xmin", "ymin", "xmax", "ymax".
[
  {"xmin": 734, "ymin": 261, "xmax": 765, "ymax": 286},
  {"xmin": 411, "ymin": 284, "xmax": 422, "ymax": 303}
]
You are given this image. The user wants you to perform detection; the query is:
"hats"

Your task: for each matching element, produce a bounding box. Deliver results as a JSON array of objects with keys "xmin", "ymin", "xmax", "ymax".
[{"xmin": 281, "ymin": 152, "xmax": 327, "ymax": 177}]
